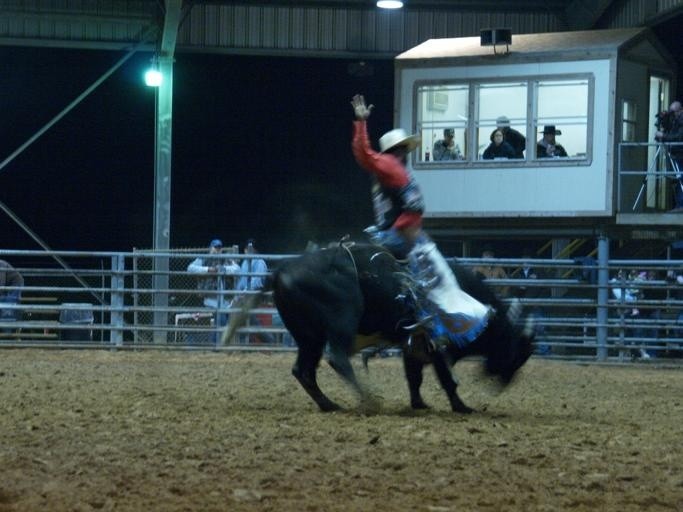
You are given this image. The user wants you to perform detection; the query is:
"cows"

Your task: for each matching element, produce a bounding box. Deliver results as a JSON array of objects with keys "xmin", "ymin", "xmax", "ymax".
[{"xmin": 221, "ymin": 238, "xmax": 542, "ymax": 415}]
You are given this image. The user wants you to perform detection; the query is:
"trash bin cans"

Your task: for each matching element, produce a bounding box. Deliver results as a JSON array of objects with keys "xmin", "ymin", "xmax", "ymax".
[{"xmin": 60, "ymin": 304, "xmax": 92, "ymax": 341}]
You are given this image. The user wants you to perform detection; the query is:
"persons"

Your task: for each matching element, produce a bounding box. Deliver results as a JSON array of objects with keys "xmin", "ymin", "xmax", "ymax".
[
  {"xmin": 233, "ymin": 238, "xmax": 275, "ymax": 344},
  {"xmin": 655, "ymin": 101, "xmax": 683, "ymax": 213},
  {"xmin": 432, "ymin": 128, "xmax": 462, "ymax": 160},
  {"xmin": 187, "ymin": 239, "xmax": 241, "ymax": 343},
  {"xmin": 472, "ymin": 242, "xmax": 552, "ymax": 352},
  {"xmin": 350, "ymin": 93, "xmax": 426, "ymax": 261},
  {"xmin": 0, "ymin": 259, "xmax": 24, "ymax": 333},
  {"xmin": 496, "ymin": 115, "xmax": 526, "ymax": 151},
  {"xmin": 610, "ymin": 267, "xmax": 683, "ymax": 359},
  {"xmin": 483, "ymin": 129, "xmax": 524, "ymax": 159},
  {"xmin": 537, "ymin": 125, "xmax": 567, "ymax": 157}
]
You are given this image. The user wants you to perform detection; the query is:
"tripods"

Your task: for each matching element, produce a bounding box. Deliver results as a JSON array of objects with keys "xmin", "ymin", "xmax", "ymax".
[{"xmin": 632, "ymin": 145, "xmax": 682, "ymax": 211}]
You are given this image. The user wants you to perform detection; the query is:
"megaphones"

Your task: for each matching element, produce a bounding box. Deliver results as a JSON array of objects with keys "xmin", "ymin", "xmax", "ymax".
[
  {"xmin": 495, "ymin": 28, "xmax": 512, "ymax": 46},
  {"xmin": 479, "ymin": 28, "xmax": 495, "ymax": 47}
]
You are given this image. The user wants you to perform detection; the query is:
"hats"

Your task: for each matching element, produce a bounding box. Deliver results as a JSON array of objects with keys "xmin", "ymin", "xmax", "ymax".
[
  {"xmin": 377, "ymin": 127, "xmax": 421, "ymax": 152},
  {"xmin": 538, "ymin": 124, "xmax": 561, "ymax": 136},
  {"xmin": 210, "ymin": 239, "xmax": 222, "ymax": 248},
  {"xmin": 444, "ymin": 128, "xmax": 456, "ymax": 137}
]
